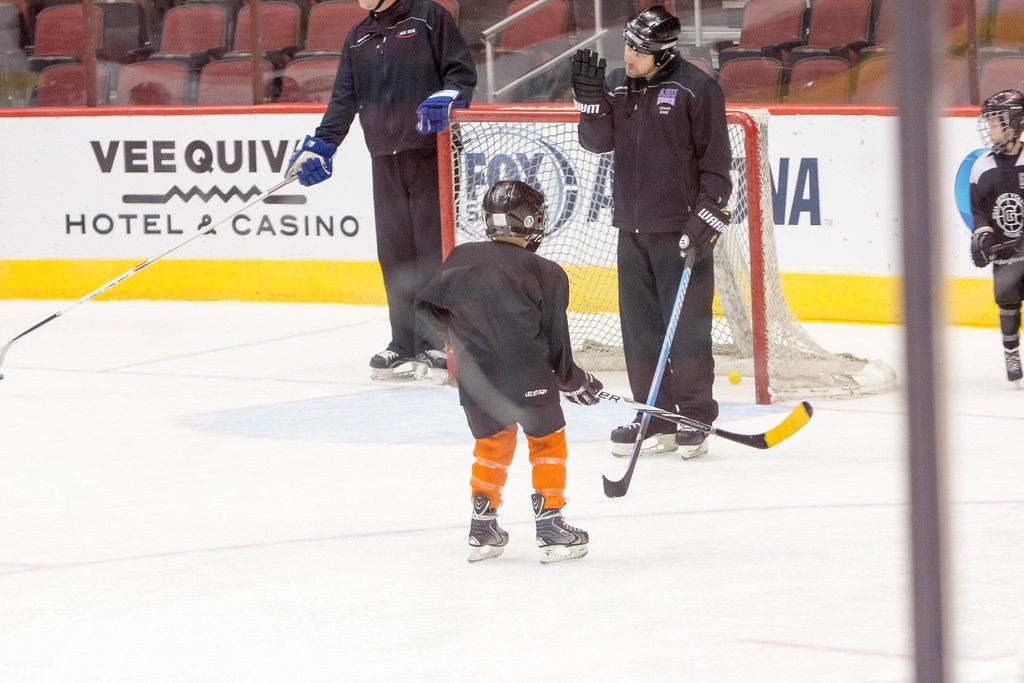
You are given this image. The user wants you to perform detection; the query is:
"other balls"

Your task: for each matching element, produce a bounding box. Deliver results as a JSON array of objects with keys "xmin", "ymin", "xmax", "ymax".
[{"xmin": 728, "ymin": 370, "xmax": 742, "ymax": 385}]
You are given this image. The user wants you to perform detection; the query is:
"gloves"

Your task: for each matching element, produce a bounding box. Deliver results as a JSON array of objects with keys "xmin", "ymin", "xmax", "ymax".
[
  {"xmin": 679, "ymin": 201, "xmax": 731, "ymax": 264},
  {"xmin": 415, "ymin": 89, "xmax": 466, "ymax": 136},
  {"xmin": 284, "ymin": 135, "xmax": 338, "ymax": 188},
  {"xmin": 571, "ymin": 48, "xmax": 612, "ymax": 120},
  {"xmin": 970, "ymin": 226, "xmax": 996, "ymax": 268},
  {"xmin": 561, "ymin": 372, "xmax": 604, "ymax": 406}
]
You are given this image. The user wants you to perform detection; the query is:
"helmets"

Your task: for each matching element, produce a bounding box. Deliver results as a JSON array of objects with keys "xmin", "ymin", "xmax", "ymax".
[
  {"xmin": 622, "ymin": 4, "xmax": 683, "ymax": 56},
  {"xmin": 482, "ymin": 180, "xmax": 549, "ymax": 252},
  {"xmin": 977, "ymin": 89, "xmax": 1023, "ymax": 155}
]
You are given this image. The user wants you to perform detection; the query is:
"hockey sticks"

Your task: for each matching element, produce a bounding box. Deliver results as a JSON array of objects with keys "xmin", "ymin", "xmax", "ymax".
[
  {"xmin": 602, "ymin": 244, "xmax": 698, "ymax": 499},
  {"xmin": 988, "ymin": 237, "xmax": 1020, "ymax": 254},
  {"xmin": 1, "ymin": 172, "xmax": 300, "ymax": 380},
  {"xmin": 592, "ymin": 389, "xmax": 813, "ymax": 449}
]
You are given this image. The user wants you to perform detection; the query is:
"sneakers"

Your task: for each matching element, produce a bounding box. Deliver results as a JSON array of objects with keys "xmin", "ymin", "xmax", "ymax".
[
  {"xmin": 531, "ymin": 493, "xmax": 590, "ymax": 564},
  {"xmin": 369, "ymin": 346, "xmax": 422, "ymax": 381},
  {"xmin": 1004, "ymin": 346, "xmax": 1023, "ymax": 391},
  {"xmin": 467, "ymin": 494, "xmax": 509, "ymax": 563},
  {"xmin": 674, "ymin": 413, "xmax": 710, "ymax": 460},
  {"xmin": 610, "ymin": 413, "xmax": 678, "ymax": 458},
  {"xmin": 413, "ymin": 348, "xmax": 450, "ymax": 386}
]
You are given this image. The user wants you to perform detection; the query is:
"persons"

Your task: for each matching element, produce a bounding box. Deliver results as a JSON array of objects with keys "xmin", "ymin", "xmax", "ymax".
[
  {"xmin": 412, "ymin": 180, "xmax": 603, "ymax": 564},
  {"xmin": 971, "ymin": 89, "xmax": 1024, "ymax": 392},
  {"xmin": 283, "ymin": 0, "xmax": 478, "ymax": 385},
  {"xmin": 571, "ymin": 4, "xmax": 733, "ymax": 460}
]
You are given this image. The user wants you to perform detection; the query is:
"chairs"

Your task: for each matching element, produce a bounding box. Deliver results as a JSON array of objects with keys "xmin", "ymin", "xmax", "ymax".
[{"xmin": 0, "ymin": 0, "xmax": 1024, "ymax": 106}]
